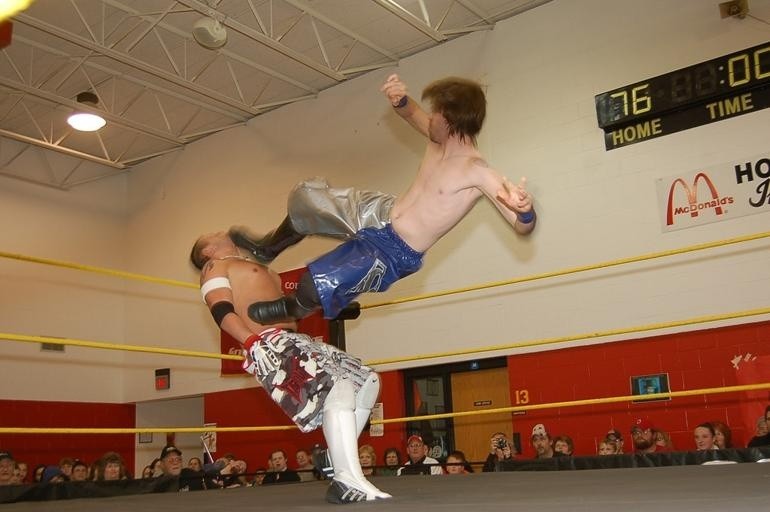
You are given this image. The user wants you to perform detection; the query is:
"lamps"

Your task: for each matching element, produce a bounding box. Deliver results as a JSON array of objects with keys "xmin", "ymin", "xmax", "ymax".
[
  {"xmin": 193, "ymin": 18, "xmax": 228, "ymax": 49},
  {"xmin": 66, "ymin": 90, "xmax": 106, "ymax": 132}
]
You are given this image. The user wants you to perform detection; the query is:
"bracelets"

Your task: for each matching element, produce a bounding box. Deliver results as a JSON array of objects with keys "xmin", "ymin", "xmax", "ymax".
[
  {"xmin": 517, "ymin": 208, "xmax": 535, "ymax": 224},
  {"xmin": 392, "ymin": 95, "xmax": 408, "ymax": 108}
]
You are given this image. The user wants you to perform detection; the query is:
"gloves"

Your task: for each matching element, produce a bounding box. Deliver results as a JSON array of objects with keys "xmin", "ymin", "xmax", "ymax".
[{"xmin": 249, "ymin": 340, "xmax": 279, "ymax": 376}]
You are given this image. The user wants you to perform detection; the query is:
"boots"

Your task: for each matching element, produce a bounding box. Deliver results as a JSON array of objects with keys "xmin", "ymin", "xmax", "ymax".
[
  {"xmin": 229, "ymin": 214, "xmax": 305, "ymax": 263},
  {"xmin": 247, "ymin": 294, "xmax": 306, "ymax": 324}
]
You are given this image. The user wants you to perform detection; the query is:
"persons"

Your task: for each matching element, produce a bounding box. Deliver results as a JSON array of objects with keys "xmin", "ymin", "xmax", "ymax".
[
  {"xmin": 228, "ymin": 72, "xmax": 537, "ymax": 326},
  {"xmin": 190, "ymin": 231, "xmax": 393, "ymax": 504},
  {"xmin": 0, "ymin": 445, "xmax": 316, "ymax": 490},
  {"xmin": 357, "ymin": 404, "xmax": 770, "ymax": 477}
]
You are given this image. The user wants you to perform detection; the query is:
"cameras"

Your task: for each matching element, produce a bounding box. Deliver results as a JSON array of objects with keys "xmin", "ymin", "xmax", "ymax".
[{"xmin": 497, "ymin": 439, "xmax": 505, "ymax": 448}]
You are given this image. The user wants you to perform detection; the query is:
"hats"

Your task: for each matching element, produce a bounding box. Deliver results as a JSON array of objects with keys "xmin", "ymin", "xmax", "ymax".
[
  {"xmin": 160, "ymin": 445, "xmax": 181, "ymax": 459},
  {"xmin": 631, "ymin": 416, "xmax": 657, "ymax": 430},
  {"xmin": 532, "ymin": 423, "xmax": 550, "ymax": 439},
  {"xmin": 408, "ymin": 435, "xmax": 422, "ymax": 443}
]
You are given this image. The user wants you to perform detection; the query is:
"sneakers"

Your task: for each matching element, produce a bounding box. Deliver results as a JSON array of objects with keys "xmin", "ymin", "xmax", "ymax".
[{"xmin": 312, "ymin": 448, "xmax": 391, "ymax": 504}]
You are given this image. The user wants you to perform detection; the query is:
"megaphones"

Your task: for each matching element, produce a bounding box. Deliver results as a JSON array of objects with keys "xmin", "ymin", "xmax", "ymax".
[{"xmin": 719, "ymin": 0, "xmax": 749, "ymax": 19}]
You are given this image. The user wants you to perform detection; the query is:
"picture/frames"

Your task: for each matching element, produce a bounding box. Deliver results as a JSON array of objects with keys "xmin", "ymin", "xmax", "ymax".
[
  {"xmin": 139, "ymin": 432, "xmax": 153, "ymax": 443},
  {"xmin": 629, "ymin": 372, "xmax": 671, "ymax": 404}
]
